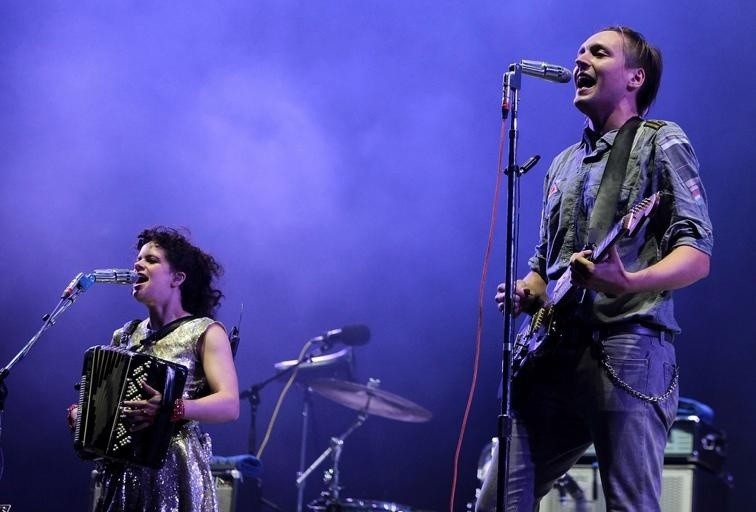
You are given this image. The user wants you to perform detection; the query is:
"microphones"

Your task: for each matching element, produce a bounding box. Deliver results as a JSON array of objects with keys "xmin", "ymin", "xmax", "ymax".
[
  {"xmin": 522, "ymin": 59, "xmax": 572, "ymax": 82},
  {"xmin": 93, "ymin": 268, "xmax": 138, "ymax": 284},
  {"xmin": 311, "ymin": 324, "xmax": 370, "ymax": 345}
]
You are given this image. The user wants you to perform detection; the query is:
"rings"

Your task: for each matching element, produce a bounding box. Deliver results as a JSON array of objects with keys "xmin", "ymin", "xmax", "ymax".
[{"xmin": 139, "ymin": 407, "xmax": 147, "ymax": 416}]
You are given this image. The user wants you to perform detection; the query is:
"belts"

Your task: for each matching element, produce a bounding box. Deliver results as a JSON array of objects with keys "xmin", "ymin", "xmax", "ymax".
[{"xmin": 597, "ymin": 321, "xmax": 680, "ymax": 344}]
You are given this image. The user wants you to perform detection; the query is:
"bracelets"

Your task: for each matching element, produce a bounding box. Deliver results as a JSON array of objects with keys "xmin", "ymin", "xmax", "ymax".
[
  {"xmin": 65, "ymin": 403, "xmax": 80, "ymax": 412},
  {"xmin": 172, "ymin": 398, "xmax": 186, "ymax": 421}
]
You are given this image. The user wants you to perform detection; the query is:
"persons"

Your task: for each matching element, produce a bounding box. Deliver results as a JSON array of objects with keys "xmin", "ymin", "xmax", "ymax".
[
  {"xmin": 66, "ymin": 227, "xmax": 241, "ymax": 511},
  {"xmin": 475, "ymin": 24, "xmax": 717, "ymax": 510}
]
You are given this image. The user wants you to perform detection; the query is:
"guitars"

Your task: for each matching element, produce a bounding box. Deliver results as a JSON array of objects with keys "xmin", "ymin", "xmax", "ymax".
[{"xmin": 497, "ymin": 192, "xmax": 660, "ymax": 405}]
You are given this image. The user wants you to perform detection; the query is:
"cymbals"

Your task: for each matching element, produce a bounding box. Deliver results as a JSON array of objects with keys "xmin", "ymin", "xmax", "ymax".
[{"xmin": 308, "ymin": 378, "xmax": 432, "ymax": 422}]
